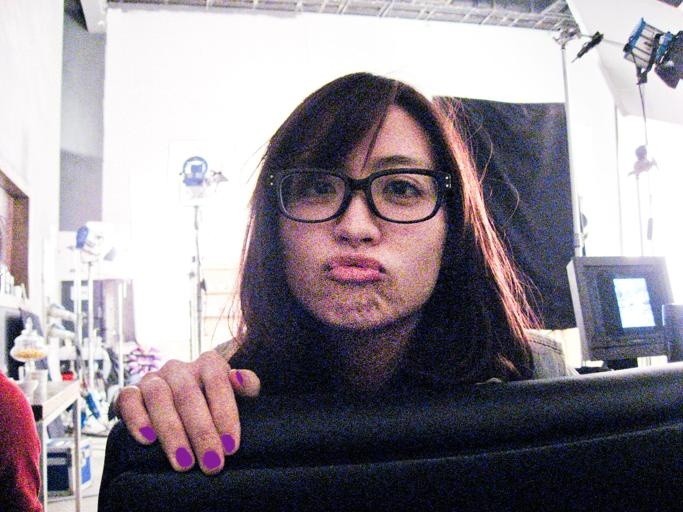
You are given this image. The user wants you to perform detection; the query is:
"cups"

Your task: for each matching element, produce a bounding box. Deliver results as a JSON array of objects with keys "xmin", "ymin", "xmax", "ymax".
[{"xmin": 31, "ymin": 369, "xmax": 49, "ymax": 400}]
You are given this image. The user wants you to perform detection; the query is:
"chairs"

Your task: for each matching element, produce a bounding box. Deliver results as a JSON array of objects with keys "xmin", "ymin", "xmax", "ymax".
[{"xmin": 102, "ymin": 372, "xmax": 681, "ymax": 512}]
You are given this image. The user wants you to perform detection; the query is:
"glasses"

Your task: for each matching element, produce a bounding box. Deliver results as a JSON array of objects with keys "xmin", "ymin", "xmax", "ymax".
[{"xmin": 274, "ymin": 166, "xmax": 452, "ymax": 224}]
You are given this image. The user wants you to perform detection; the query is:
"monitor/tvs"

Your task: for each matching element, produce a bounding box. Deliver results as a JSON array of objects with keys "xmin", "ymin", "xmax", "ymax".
[{"xmin": 564, "ymin": 255, "xmax": 673, "ymax": 369}]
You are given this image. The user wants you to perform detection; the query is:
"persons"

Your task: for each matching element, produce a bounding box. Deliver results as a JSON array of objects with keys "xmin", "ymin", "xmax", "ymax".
[
  {"xmin": 108, "ymin": 72, "xmax": 581, "ymax": 477},
  {"xmin": 0, "ymin": 368, "xmax": 44, "ymax": 512}
]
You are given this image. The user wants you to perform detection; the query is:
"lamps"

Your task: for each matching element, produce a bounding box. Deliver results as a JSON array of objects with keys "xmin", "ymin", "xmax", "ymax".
[{"xmin": 616, "ymin": 16, "xmax": 682, "ymax": 90}]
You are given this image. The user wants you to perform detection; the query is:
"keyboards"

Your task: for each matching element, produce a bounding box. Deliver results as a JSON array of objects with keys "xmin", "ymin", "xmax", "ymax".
[{"xmin": 573, "ymin": 366, "xmax": 611, "ymax": 375}]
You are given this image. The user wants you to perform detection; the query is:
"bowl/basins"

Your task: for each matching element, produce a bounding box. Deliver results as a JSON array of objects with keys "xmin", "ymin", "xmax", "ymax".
[{"xmin": 17, "ymin": 379, "xmax": 38, "ymax": 399}]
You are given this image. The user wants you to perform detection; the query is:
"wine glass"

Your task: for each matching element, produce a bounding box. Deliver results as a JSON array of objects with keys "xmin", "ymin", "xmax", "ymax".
[{"xmin": 12, "ymin": 333, "xmax": 49, "ymax": 373}]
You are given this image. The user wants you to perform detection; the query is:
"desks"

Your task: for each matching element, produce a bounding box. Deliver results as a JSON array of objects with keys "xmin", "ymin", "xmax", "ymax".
[{"xmin": 10, "ymin": 380, "xmax": 83, "ymax": 511}]
